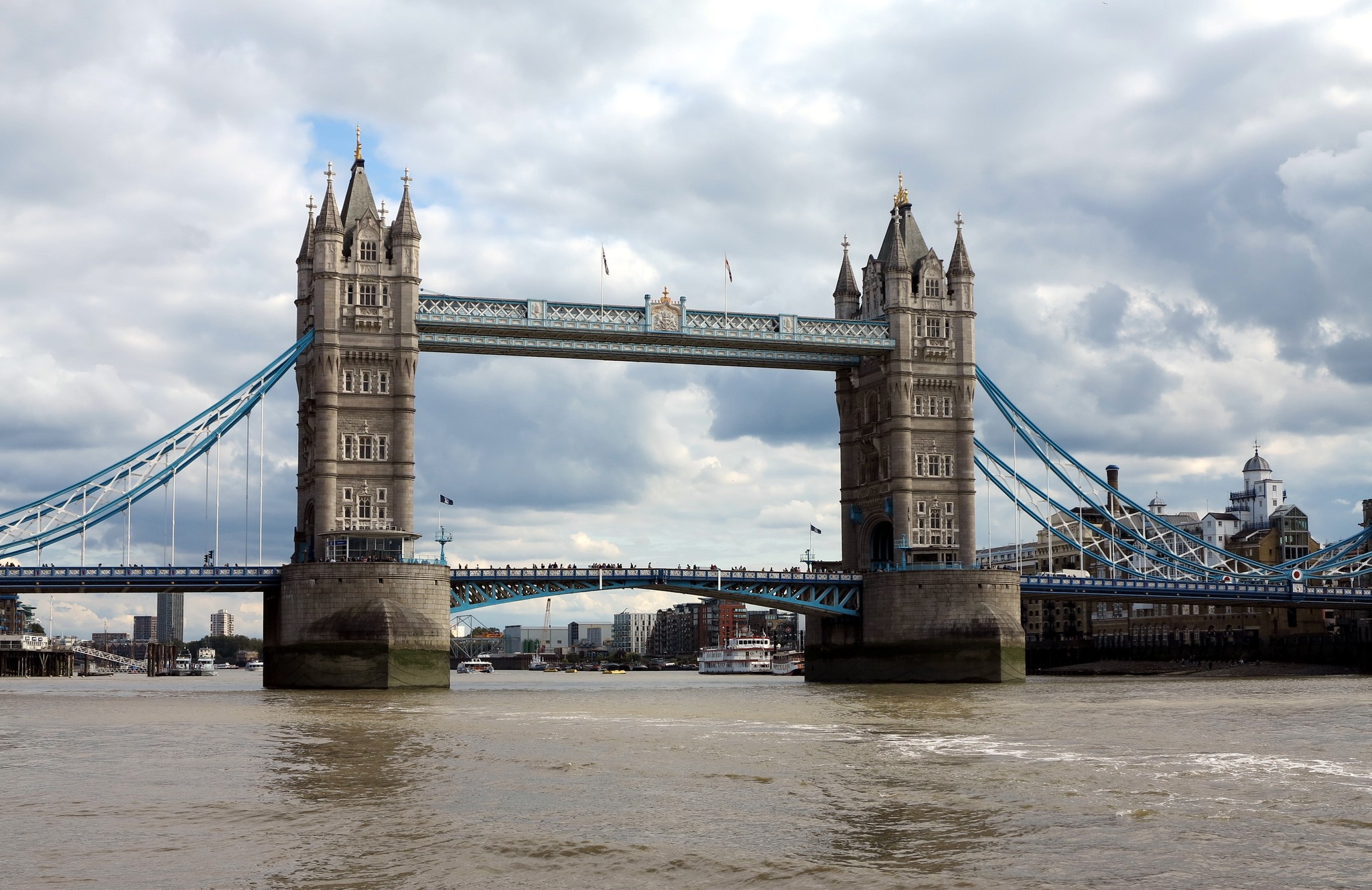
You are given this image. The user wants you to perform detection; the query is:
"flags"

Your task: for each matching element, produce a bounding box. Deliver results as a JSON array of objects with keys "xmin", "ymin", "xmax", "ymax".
[
  {"xmin": 726, "ymin": 255, "xmax": 732, "ymax": 282},
  {"xmin": 440, "ymin": 494, "xmax": 453, "ymax": 505},
  {"xmin": 603, "ymin": 248, "xmax": 609, "ymax": 275},
  {"xmin": 811, "ymin": 525, "xmax": 821, "ymax": 534}
]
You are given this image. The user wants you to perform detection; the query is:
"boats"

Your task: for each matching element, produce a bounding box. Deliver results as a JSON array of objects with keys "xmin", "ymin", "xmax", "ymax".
[
  {"xmin": 171, "ymin": 645, "xmax": 194, "ymax": 676},
  {"xmin": 544, "ymin": 666, "xmax": 561, "ymax": 671},
  {"xmin": 697, "ymin": 617, "xmax": 774, "ymax": 674},
  {"xmin": 565, "ymin": 657, "xmax": 698, "ymax": 671},
  {"xmin": 194, "ymin": 637, "xmax": 218, "ymax": 676},
  {"xmin": 528, "ymin": 642, "xmax": 547, "ymax": 671},
  {"xmin": 771, "ymin": 650, "xmax": 805, "ymax": 675},
  {"xmin": 214, "ymin": 662, "xmax": 238, "ymax": 669},
  {"xmin": 249, "ymin": 660, "xmax": 264, "ymax": 671},
  {"xmin": 457, "ymin": 658, "xmax": 494, "ymax": 674}
]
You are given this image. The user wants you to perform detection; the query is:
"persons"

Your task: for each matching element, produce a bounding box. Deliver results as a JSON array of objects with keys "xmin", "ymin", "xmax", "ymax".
[
  {"xmin": 0, "ymin": 555, "xmax": 887, "ymax": 574},
  {"xmin": 1180, "ymin": 655, "xmax": 1260, "ymax": 670},
  {"xmin": 979, "ymin": 564, "xmax": 1372, "ymax": 590}
]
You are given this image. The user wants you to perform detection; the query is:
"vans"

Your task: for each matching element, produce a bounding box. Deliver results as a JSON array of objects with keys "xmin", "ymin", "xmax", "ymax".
[{"xmin": 1034, "ymin": 572, "xmax": 1068, "ymax": 578}]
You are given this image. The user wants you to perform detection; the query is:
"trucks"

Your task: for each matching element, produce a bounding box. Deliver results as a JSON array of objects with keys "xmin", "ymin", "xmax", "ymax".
[{"xmin": 1055, "ymin": 569, "xmax": 1091, "ymax": 578}]
